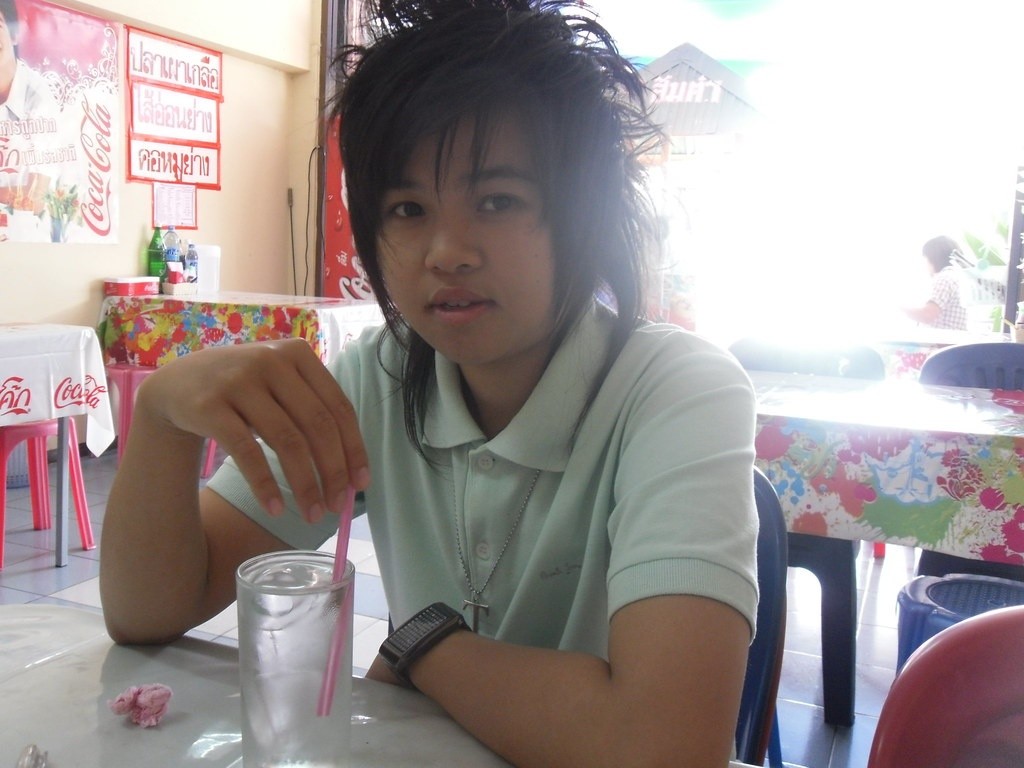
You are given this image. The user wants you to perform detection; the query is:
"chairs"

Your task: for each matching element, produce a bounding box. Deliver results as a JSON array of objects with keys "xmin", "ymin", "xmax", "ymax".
[
  {"xmin": 736, "ymin": 465, "xmax": 787, "ymax": 767},
  {"xmin": 787, "ymin": 531, "xmax": 860, "ymax": 728},
  {"xmin": 865, "ymin": 604, "xmax": 1023, "ymax": 768},
  {"xmin": 919, "ymin": 342, "xmax": 1024, "ymax": 581}
]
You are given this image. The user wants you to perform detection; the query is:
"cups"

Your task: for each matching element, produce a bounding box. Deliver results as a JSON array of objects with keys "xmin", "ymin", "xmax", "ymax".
[{"xmin": 235, "ymin": 551, "xmax": 356, "ymax": 767}]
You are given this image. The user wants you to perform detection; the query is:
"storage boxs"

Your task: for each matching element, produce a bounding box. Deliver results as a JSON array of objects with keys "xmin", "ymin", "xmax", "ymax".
[
  {"xmin": 103, "ymin": 277, "xmax": 161, "ymax": 296},
  {"xmin": 162, "ymin": 282, "xmax": 198, "ymax": 295}
]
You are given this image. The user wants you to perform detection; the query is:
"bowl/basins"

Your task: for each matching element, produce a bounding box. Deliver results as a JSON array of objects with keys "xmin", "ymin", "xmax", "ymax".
[{"xmin": 105, "ymin": 275, "xmax": 161, "ymax": 295}]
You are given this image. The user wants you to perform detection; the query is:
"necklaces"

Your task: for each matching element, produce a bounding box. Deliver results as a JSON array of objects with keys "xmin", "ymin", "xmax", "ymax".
[{"xmin": 453, "ymin": 466, "xmax": 541, "ymax": 634}]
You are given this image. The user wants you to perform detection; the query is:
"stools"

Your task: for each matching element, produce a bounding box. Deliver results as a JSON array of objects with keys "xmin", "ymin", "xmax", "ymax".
[
  {"xmin": 1, "ymin": 417, "xmax": 96, "ymax": 569},
  {"xmin": 91, "ymin": 362, "xmax": 218, "ymax": 478}
]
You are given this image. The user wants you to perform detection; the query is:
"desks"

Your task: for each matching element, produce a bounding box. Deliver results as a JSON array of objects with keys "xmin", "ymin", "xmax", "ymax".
[
  {"xmin": 748, "ymin": 368, "xmax": 1024, "ymax": 567},
  {"xmin": 880, "ymin": 325, "xmax": 1013, "ymax": 381},
  {"xmin": 0, "ymin": 603, "xmax": 509, "ymax": 768},
  {"xmin": 0, "ymin": 322, "xmax": 118, "ymax": 568},
  {"xmin": 94, "ymin": 290, "xmax": 385, "ymax": 366}
]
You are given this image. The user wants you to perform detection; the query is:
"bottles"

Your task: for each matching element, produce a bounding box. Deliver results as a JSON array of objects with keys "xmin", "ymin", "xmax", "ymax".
[
  {"xmin": 148, "ymin": 222, "xmax": 168, "ymax": 292},
  {"xmin": 164, "ymin": 226, "xmax": 198, "ymax": 283}
]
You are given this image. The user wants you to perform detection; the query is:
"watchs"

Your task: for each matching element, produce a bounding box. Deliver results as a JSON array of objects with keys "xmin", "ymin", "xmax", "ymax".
[{"xmin": 379, "ymin": 602, "xmax": 473, "ymax": 688}]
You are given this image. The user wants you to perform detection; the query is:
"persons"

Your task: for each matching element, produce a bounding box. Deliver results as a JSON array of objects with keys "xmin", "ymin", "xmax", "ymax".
[
  {"xmin": 900, "ymin": 235, "xmax": 980, "ymax": 329},
  {"xmin": 0, "ymin": 1, "xmax": 67, "ymax": 218},
  {"xmin": 100, "ymin": 1, "xmax": 761, "ymax": 768}
]
